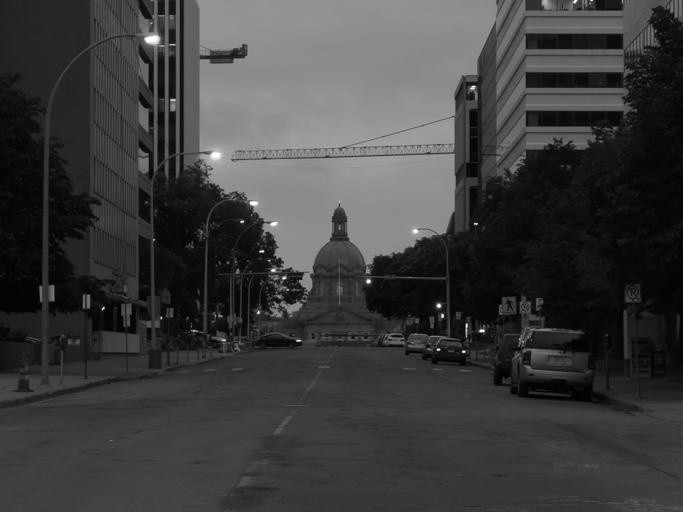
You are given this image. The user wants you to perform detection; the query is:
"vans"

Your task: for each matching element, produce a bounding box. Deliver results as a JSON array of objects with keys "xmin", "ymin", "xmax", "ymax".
[{"xmin": 510, "ymin": 328, "xmax": 596, "ymax": 401}]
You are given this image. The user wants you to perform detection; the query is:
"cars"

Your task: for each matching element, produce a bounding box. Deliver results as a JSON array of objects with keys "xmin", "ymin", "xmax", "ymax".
[
  {"xmin": 377, "ymin": 333, "xmax": 405, "ymax": 347},
  {"xmin": 174, "ymin": 329, "xmax": 227, "ymax": 352},
  {"xmin": 252, "ymin": 333, "xmax": 303, "ymax": 349},
  {"xmin": 404, "ymin": 333, "xmax": 467, "ymax": 367},
  {"xmin": 491, "ymin": 333, "xmax": 523, "ymax": 384}
]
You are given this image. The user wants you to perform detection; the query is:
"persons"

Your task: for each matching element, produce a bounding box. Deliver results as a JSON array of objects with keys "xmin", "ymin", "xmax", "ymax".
[{"xmin": 494, "ymin": 344, "xmax": 504, "ymax": 384}]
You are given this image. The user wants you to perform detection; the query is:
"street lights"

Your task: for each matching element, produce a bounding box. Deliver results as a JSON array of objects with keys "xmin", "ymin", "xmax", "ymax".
[
  {"xmin": 41, "ymin": 30, "xmax": 162, "ymax": 387},
  {"xmin": 411, "ymin": 227, "xmax": 451, "ymax": 338},
  {"xmin": 201, "ymin": 197, "xmax": 279, "ymax": 358},
  {"xmin": 147, "ymin": 149, "xmax": 221, "ymax": 370}
]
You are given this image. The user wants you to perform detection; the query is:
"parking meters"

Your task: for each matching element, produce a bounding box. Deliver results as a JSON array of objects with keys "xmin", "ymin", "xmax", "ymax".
[{"xmin": 55, "ymin": 334, "xmax": 69, "ymax": 385}]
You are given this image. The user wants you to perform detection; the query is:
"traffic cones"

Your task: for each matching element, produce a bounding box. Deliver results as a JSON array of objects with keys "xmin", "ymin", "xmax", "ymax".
[{"xmin": 13, "ymin": 357, "xmax": 37, "ymax": 392}]
